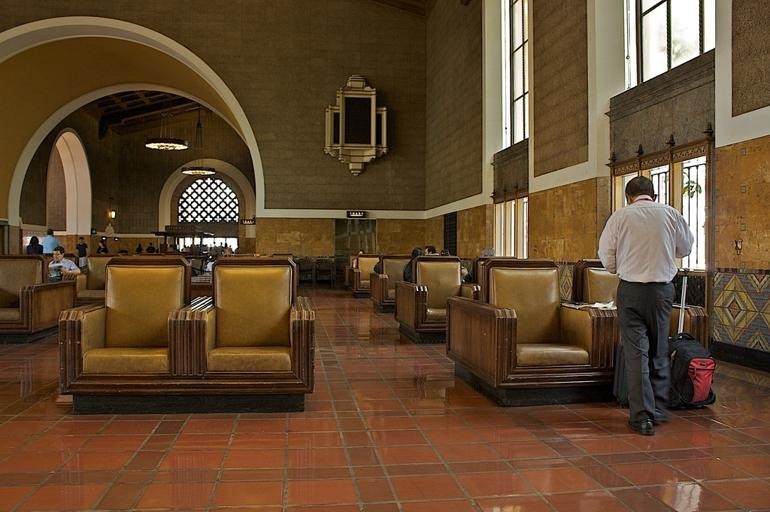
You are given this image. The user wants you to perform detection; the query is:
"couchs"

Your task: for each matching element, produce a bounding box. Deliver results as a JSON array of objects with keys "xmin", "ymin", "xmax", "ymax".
[
  {"xmin": 302, "ymin": 252, "xmax": 381, "ymax": 298},
  {"xmin": 74, "ymin": 255, "xmax": 120, "ymax": 305},
  {"xmin": 395, "ymin": 255, "xmax": 578, "ymax": 344},
  {"xmin": 191, "ymin": 256, "xmax": 317, "ymax": 398},
  {"xmin": 45, "ymin": 254, "xmax": 78, "ymax": 309},
  {"xmin": 59, "ymin": 253, "xmax": 191, "ymax": 397},
  {"xmin": 446, "ymin": 258, "xmax": 607, "ymax": 407},
  {"xmin": 368, "ymin": 256, "xmax": 416, "ymax": 313},
  {"xmin": 0, "ymin": 253, "xmax": 77, "ymax": 343},
  {"xmin": 572, "ymin": 259, "xmax": 711, "ymax": 401}
]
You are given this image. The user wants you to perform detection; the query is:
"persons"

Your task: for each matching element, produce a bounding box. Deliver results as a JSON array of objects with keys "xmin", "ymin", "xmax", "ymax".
[
  {"xmin": 595, "ymin": 175, "xmax": 695, "ymax": 437},
  {"xmin": 353, "ymin": 245, "xmax": 497, "ymax": 285},
  {"xmin": 26, "ymin": 228, "xmax": 234, "ymax": 281}
]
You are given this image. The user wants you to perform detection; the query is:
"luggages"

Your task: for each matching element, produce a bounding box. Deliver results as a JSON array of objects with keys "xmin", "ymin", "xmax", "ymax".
[
  {"xmin": 670, "ymin": 276, "xmax": 716, "ymax": 410},
  {"xmin": 612, "ymin": 346, "xmax": 630, "ymax": 408}
]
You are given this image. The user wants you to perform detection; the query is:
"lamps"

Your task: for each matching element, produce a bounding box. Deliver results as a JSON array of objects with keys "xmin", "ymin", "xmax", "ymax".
[
  {"xmin": 110, "ymin": 209, "xmax": 117, "ymax": 220},
  {"xmin": 145, "ymin": 112, "xmax": 191, "ymax": 152},
  {"xmin": 179, "ymin": 108, "xmax": 217, "ymax": 176}
]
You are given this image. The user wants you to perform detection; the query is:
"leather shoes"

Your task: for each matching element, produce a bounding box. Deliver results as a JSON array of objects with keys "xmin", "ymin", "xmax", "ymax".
[
  {"xmin": 628, "ymin": 418, "xmax": 654, "ymax": 436},
  {"xmin": 655, "ymin": 408, "xmax": 670, "ymax": 423}
]
